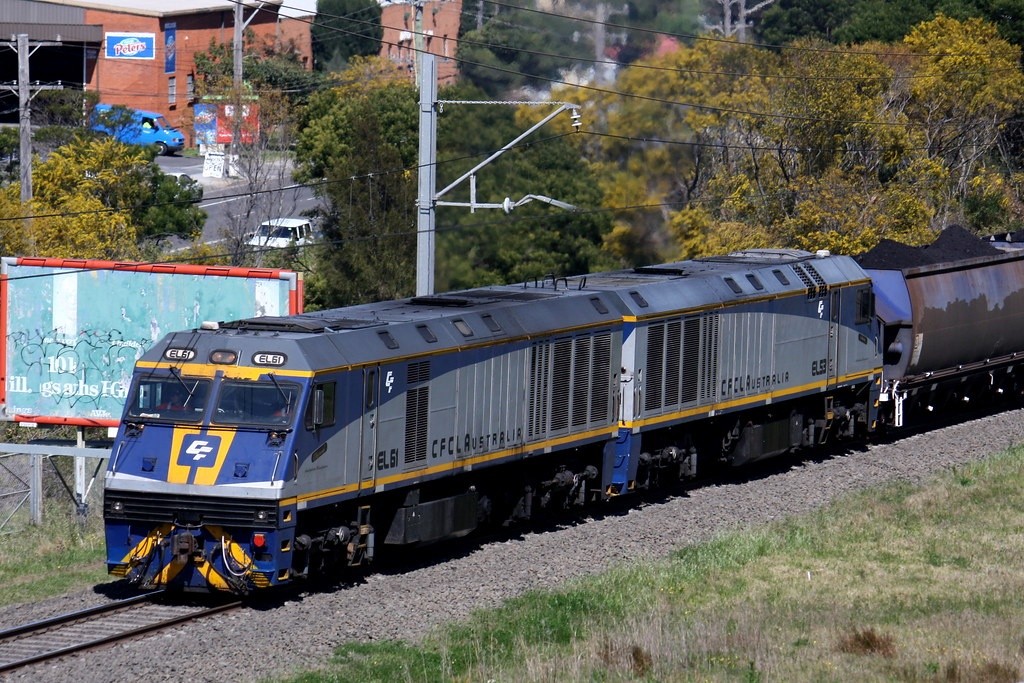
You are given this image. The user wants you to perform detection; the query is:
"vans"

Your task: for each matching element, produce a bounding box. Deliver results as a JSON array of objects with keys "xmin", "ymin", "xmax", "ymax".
[{"xmin": 87, "ymin": 103, "xmax": 185, "ymax": 157}]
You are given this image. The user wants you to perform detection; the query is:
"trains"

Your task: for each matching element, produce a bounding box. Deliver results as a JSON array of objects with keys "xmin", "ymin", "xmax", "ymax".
[{"xmin": 103, "ymin": 247, "xmax": 1024, "ymax": 601}]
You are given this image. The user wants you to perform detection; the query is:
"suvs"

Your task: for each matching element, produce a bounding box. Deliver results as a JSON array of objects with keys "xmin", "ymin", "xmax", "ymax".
[{"xmin": 243, "ymin": 219, "xmax": 315, "ymax": 260}]
